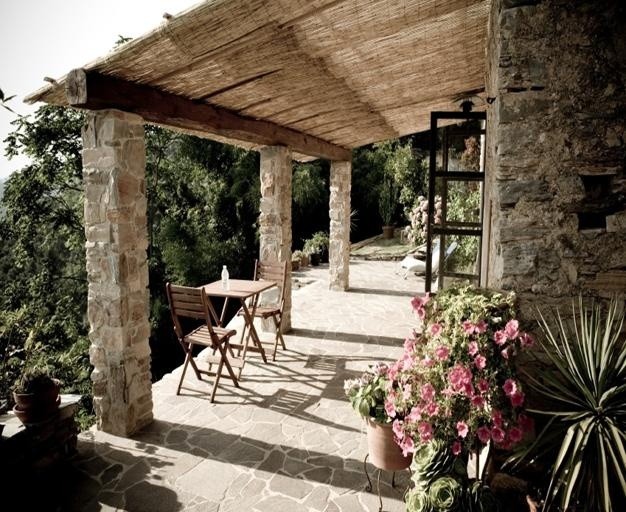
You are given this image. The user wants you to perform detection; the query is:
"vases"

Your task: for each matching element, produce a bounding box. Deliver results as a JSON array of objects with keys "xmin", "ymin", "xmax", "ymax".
[
  {"xmin": 366, "ymin": 415, "xmax": 411, "ymax": 471},
  {"xmin": 476, "ymin": 287, "xmax": 516, "ymax": 309}
]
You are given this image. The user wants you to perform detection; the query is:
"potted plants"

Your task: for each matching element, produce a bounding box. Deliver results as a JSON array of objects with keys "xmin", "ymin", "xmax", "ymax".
[
  {"xmin": 378, "ymin": 176, "xmax": 401, "ymax": 239},
  {"xmin": 13, "ymin": 365, "xmax": 61, "ymax": 428},
  {"xmin": 502, "ymin": 292, "xmax": 626, "ymax": 512},
  {"xmin": 292, "ymin": 231, "xmax": 329, "ymax": 269}
]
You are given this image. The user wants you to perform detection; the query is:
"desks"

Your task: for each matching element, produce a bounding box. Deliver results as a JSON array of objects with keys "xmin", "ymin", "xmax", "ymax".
[
  {"xmin": 0, "ymin": 394, "xmax": 82, "ymax": 461},
  {"xmin": 197, "ymin": 279, "xmax": 277, "ymax": 381}
]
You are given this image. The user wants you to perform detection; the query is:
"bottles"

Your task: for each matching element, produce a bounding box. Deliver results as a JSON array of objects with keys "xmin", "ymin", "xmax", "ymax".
[{"xmin": 220, "ymin": 265, "xmax": 228, "ymax": 290}]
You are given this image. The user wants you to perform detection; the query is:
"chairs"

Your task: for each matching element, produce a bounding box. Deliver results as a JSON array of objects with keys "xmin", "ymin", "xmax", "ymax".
[
  {"xmin": 237, "ymin": 258, "xmax": 288, "ymax": 361},
  {"xmin": 166, "ymin": 283, "xmax": 240, "ymax": 402},
  {"xmin": 396, "ymin": 241, "xmax": 457, "ymax": 279}
]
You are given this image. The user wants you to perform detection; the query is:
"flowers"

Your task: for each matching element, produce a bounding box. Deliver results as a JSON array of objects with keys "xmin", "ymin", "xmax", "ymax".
[
  {"xmin": 405, "ymin": 194, "xmax": 450, "ymax": 243},
  {"xmin": 388, "ymin": 281, "xmax": 532, "ymax": 512},
  {"xmin": 343, "ymin": 361, "xmax": 388, "ymax": 422}
]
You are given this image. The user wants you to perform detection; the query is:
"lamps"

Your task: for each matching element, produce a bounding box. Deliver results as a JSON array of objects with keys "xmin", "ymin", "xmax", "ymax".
[{"xmin": 453, "ymin": 93, "xmax": 485, "ymax": 111}]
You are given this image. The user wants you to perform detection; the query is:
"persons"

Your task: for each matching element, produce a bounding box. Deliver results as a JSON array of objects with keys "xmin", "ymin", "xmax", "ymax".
[{"xmin": 0, "ymin": 371, "xmax": 90, "ymax": 512}]
